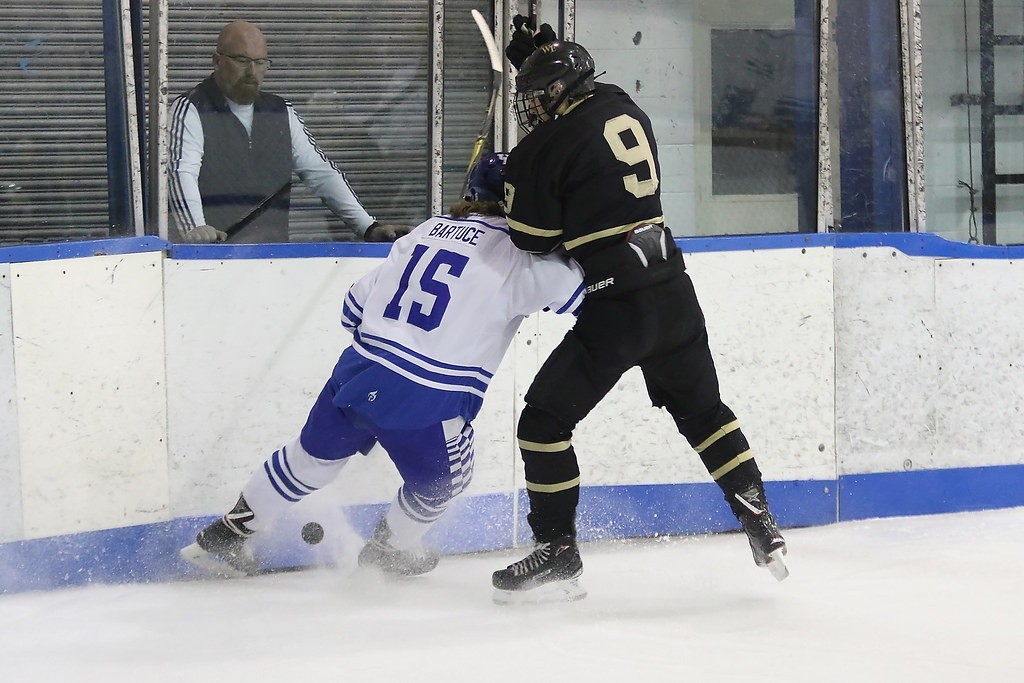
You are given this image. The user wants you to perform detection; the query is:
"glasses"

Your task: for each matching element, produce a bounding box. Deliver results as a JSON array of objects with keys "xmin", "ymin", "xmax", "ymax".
[{"xmin": 216, "ymin": 53, "xmax": 273, "ymax": 71}]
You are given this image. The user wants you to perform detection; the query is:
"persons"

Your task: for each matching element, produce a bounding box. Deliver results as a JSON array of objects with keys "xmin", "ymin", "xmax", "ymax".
[
  {"xmin": 168, "ymin": 22, "xmax": 413, "ymax": 243},
  {"xmin": 491, "ymin": 41, "xmax": 790, "ymax": 606},
  {"xmin": 181, "ymin": 153, "xmax": 585, "ymax": 577}
]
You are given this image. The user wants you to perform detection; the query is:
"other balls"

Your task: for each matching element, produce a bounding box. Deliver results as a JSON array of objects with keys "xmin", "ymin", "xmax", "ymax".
[{"xmin": 300, "ymin": 521, "xmax": 325, "ymax": 546}]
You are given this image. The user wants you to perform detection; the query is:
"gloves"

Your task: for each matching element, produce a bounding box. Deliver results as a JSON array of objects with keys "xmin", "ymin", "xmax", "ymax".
[{"xmin": 504, "ymin": 14, "xmax": 559, "ymax": 70}]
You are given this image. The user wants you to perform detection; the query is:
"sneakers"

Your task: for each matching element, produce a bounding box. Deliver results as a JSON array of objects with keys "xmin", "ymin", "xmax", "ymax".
[
  {"xmin": 489, "ymin": 511, "xmax": 588, "ymax": 602},
  {"xmin": 178, "ymin": 492, "xmax": 257, "ymax": 579},
  {"xmin": 356, "ymin": 516, "xmax": 448, "ymax": 583},
  {"xmin": 724, "ymin": 479, "xmax": 789, "ymax": 582}
]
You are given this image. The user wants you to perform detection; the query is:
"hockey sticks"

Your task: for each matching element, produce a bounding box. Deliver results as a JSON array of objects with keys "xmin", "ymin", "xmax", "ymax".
[
  {"xmin": 527, "ymin": 0, "xmax": 537, "ymax": 129},
  {"xmin": 456, "ymin": 7, "xmax": 506, "ymax": 216}
]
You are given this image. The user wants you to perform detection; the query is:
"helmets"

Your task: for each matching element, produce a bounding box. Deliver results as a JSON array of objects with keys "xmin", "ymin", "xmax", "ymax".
[
  {"xmin": 463, "ymin": 152, "xmax": 510, "ymax": 207},
  {"xmin": 512, "ymin": 39, "xmax": 596, "ymax": 136}
]
